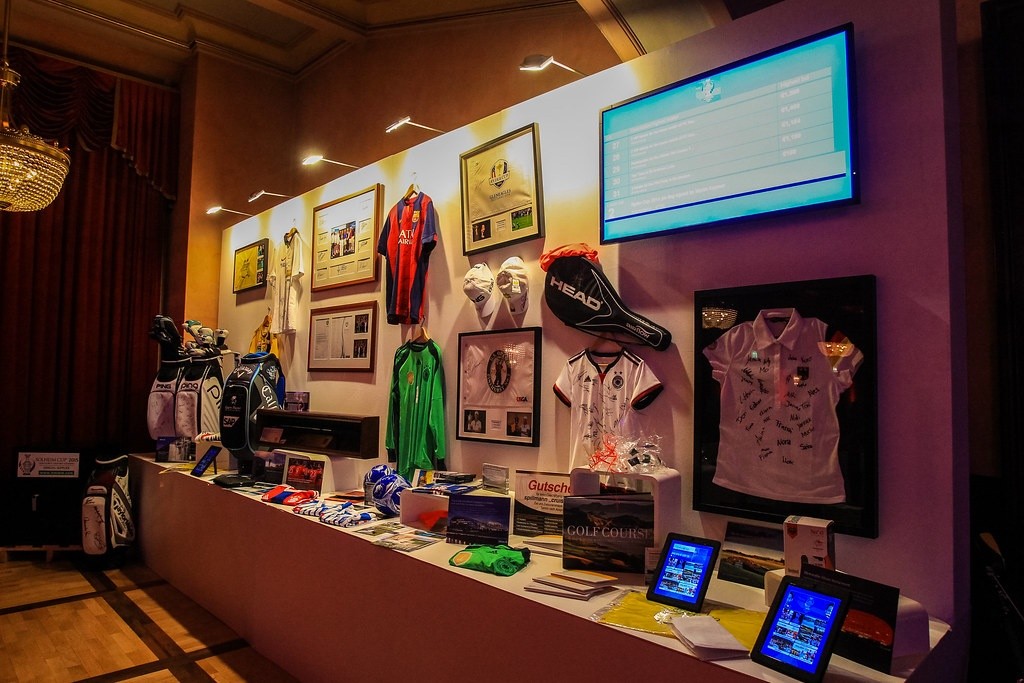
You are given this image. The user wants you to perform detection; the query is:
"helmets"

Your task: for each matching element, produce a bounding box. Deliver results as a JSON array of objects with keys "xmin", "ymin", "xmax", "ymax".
[
  {"xmin": 364, "ymin": 463, "xmax": 397, "ymax": 502},
  {"xmin": 372, "ymin": 474, "xmax": 414, "ymax": 516}
]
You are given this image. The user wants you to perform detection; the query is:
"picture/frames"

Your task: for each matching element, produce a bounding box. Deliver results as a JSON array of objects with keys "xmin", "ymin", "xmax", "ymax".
[
  {"xmin": 646, "ymin": 531, "xmax": 721, "ymax": 614},
  {"xmin": 307, "ymin": 300, "xmax": 377, "ymax": 372},
  {"xmin": 459, "ymin": 122, "xmax": 547, "ymax": 256},
  {"xmin": 695, "ymin": 275, "xmax": 880, "ymax": 541},
  {"xmin": 233, "ymin": 238, "xmax": 269, "ymax": 294},
  {"xmin": 310, "ymin": 183, "xmax": 380, "ymax": 293},
  {"xmin": 190, "ymin": 445, "xmax": 223, "ymax": 477},
  {"xmin": 455, "ymin": 326, "xmax": 543, "ymax": 449},
  {"xmin": 751, "ymin": 574, "xmax": 851, "ymax": 683}
]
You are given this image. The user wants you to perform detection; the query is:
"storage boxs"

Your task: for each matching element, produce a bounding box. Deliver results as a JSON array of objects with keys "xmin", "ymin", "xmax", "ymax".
[
  {"xmin": 782, "ymin": 516, "xmax": 836, "ymax": 576},
  {"xmin": 195, "ymin": 441, "xmax": 238, "ymax": 470}
]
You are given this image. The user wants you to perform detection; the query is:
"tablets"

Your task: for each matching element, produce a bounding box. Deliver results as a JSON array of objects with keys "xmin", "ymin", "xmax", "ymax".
[
  {"xmin": 750, "ymin": 575, "xmax": 853, "ymax": 683},
  {"xmin": 646, "ymin": 532, "xmax": 721, "ymax": 614}
]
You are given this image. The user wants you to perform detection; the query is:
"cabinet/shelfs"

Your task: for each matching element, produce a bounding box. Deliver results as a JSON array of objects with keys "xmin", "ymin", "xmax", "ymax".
[{"xmin": 255, "ymin": 409, "xmax": 379, "ymax": 459}]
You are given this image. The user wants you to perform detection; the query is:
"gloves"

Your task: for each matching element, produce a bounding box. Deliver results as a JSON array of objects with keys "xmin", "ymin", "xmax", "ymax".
[{"xmin": 261, "ymin": 484, "xmax": 371, "ymax": 528}]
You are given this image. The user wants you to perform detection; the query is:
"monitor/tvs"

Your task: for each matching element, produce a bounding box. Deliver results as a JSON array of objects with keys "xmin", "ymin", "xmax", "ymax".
[{"xmin": 598, "ymin": 20, "xmax": 862, "ymax": 245}]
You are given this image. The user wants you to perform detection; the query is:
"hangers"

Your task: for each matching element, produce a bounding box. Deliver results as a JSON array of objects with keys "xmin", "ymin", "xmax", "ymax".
[
  {"xmin": 589, "ymin": 330, "xmax": 621, "ymax": 367},
  {"xmin": 411, "ymin": 315, "xmax": 430, "ymax": 344},
  {"xmin": 264, "ymin": 306, "xmax": 271, "ymax": 325},
  {"xmin": 405, "ymin": 172, "xmax": 419, "ymax": 199},
  {"xmin": 287, "ymin": 219, "xmax": 296, "ymax": 237}
]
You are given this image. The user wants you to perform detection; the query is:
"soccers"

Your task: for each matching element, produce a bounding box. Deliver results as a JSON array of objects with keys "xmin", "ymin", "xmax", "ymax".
[{"xmin": 363, "ymin": 464, "xmax": 414, "ymax": 515}]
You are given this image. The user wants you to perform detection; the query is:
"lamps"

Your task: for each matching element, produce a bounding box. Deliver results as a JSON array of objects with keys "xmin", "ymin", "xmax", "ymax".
[
  {"xmin": 206, "ymin": 206, "xmax": 253, "ymax": 218},
  {"xmin": 303, "ymin": 154, "xmax": 360, "ymax": 170},
  {"xmin": 385, "ymin": 116, "xmax": 445, "ymax": 134},
  {"xmin": 0, "ymin": 0, "xmax": 71, "ymax": 212},
  {"xmin": 518, "ymin": 55, "xmax": 587, "ymax": 78},
  {"xmin": 247, "ymin": 189, "xmax": 294, "ymax": 202}
]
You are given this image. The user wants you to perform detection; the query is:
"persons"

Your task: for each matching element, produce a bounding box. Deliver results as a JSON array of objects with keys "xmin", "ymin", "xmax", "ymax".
[
  {"xmin": 466, "ymin": 411, "xmax": 482, "ymax": 432},
  {"xmin": 330, "ymin": 224, "xmax": 354, "ymax": 258},
  {"xmin": 510, "ymin": 416, "xmax": 530, "ymax": 434}
]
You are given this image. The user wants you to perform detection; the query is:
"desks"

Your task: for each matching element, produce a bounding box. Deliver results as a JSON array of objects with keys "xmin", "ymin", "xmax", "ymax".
[
  {"xmin": 764, "ymin": 568, "xmax": 930, "ymax": 658},
  {"xmin": 399, "ymin": 489, "xmax": 515, "ymax": 535},
  {"xmin": 569, "ymin": 465, "xmax": 681, "ymax": 547}
]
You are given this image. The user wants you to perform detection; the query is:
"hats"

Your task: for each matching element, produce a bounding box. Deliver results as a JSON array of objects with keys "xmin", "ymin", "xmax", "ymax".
[
  {"xmin": 464, "ymin": 262, "xmax": 494, "ymax": 317},
  {"xmin": 496, "ymin": 256, "xmax": 529, "ymax": 314}
]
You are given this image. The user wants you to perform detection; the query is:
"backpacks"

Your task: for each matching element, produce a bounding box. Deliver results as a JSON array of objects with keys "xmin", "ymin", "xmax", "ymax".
[{"xmin": 219, "ymin": 351, "xmax": 286, "ymax": 462}]
